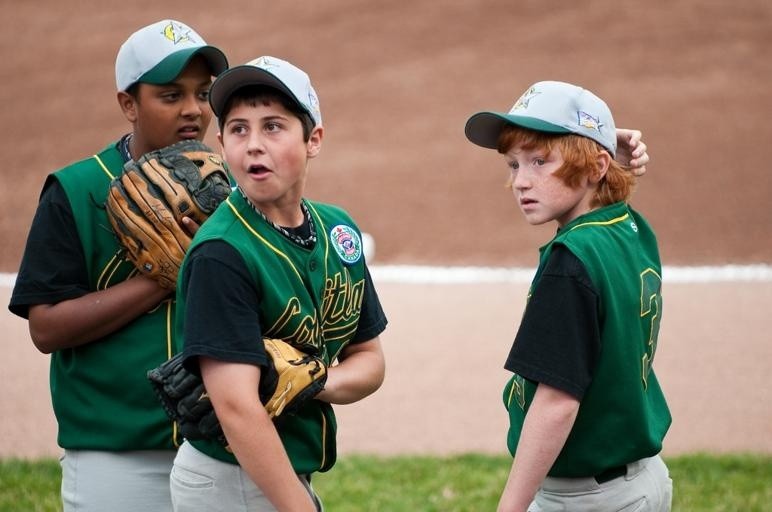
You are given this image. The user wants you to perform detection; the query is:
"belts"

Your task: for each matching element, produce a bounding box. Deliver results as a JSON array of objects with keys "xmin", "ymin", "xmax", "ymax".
[{"xmin": 595, "ymin": 464, "xmax": 627, "ymax": 484}]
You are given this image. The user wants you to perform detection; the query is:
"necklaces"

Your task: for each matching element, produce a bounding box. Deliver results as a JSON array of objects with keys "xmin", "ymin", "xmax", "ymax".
[
  {"xmin": 236, "ymin": 186, "xmax": 316, "ymax": 246},
  {"xmin": 122, "ymin": 130, "xmax": 137, "ymax": 163}
]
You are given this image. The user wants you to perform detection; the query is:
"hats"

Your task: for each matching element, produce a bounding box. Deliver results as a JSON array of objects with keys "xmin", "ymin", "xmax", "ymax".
[
  {"xmin": 464, "ymin": 79, "xmax": 619, "ymax": 157},
  {"xmin": 115, "ymin": 19, "xmax": 229, "ymax": 92},
  {"xmin": 208, "ymin": 56, "xmax": 322, "ymax": 126}
]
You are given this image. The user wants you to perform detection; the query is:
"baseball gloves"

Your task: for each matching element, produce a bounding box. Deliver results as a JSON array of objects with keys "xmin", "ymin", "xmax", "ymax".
[
  {"xmin": 88, "ymin": 140, "xmax": 233, "ymax": 292},
  {"xmin": 146, "ymin": 336, "xmax": 328, "ymax": 453}
]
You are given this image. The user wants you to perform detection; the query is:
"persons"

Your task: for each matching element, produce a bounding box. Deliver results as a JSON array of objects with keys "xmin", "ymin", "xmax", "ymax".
[
  {"xmin": 465, "ymin": 81, "xmax": 673, "ymax": 512},
  {"xmin": 8, "ymin": 20, "xmax": 229, "ymax": 512},
  {"xmin": 147, "ymin": 56, "xmax": 388, "ymax": 512}
]
